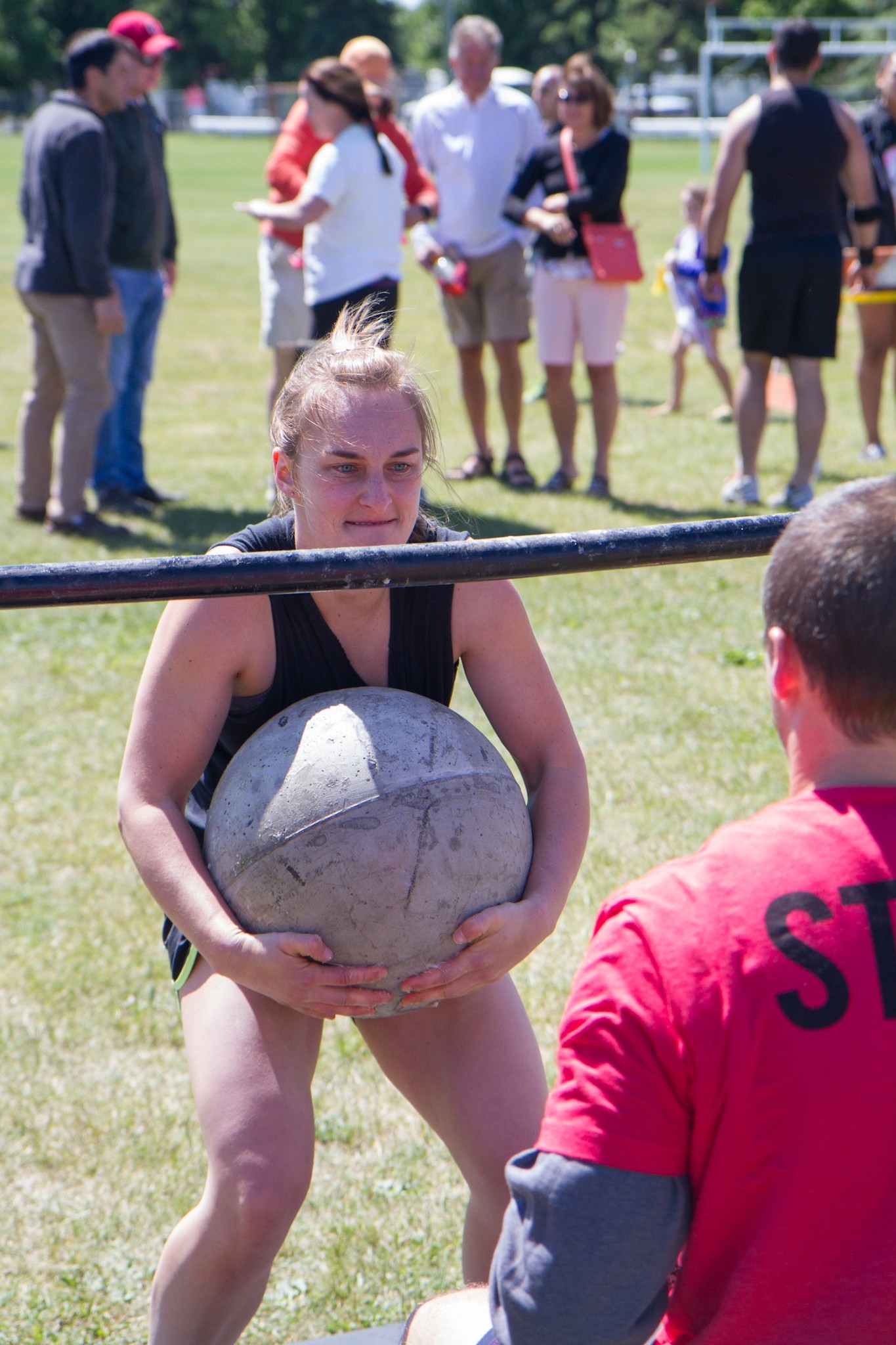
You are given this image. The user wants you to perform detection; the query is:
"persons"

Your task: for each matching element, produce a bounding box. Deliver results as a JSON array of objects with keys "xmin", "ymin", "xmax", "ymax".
[
  {"xmin": 118, "ymin": 292, "xmax": 592, "ymax": 1345},
  {"xmin": 233, "ymin": 16, "xmax": 641, "ymax": 500},
  {"xmin": 406, "ymin": 473, "xmax": 896, "ymax": 1345},
  {"xmin": 653, "ymin": 17, "xmax": 896, "ymax": 508},
  {"xmin": 14, "ymin": 10, "xmax": 184, "ymax": 538}
]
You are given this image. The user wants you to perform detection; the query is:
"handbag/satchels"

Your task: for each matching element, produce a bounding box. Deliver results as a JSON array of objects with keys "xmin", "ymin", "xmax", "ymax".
[{"xmin": 583, "ymin": 223, "xmax": 644, "ymax": 285}]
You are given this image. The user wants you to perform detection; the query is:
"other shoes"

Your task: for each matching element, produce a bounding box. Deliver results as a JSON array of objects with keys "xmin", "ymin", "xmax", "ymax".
[
  {"xmin": 108, "ymin": 489, "xmax": 180, "ymax": 515},
  {"xmin": 47, "ymin": 513, "xmax": 124, "ymax": 544},
  {"xmin": 541, "ymin": 470, "xmax": 571, "ymax": 494},
  {"xmin": 723, "ymin": 476, "xmax": 754, "ymax": 504},
  {"xmin": 861, "ymin": 442, "xmax": 883, "ymax": 460},
  {"xmin": 765, "ymin": 485, "xmax": 812, "ymax": 512},
  {"xmin": 586, "ymin": 473, "xmax": 608, "ymax": 500}
]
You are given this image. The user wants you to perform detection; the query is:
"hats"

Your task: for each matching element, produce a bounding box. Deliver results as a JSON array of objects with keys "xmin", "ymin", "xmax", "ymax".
[{"xmin": 110, "ymin": 11, "xmax": 180, "ymax": 57}]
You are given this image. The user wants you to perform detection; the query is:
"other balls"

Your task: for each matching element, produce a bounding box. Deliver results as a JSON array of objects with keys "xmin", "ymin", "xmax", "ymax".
[{"xmin": 201, "ymin": 684, "xmax": 536, "ymax": 1019}]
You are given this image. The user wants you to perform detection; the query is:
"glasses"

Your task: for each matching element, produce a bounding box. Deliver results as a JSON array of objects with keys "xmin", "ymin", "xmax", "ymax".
[{"xmin": 555, "ymin": 91, "xmax": 591, "ymax": 107}]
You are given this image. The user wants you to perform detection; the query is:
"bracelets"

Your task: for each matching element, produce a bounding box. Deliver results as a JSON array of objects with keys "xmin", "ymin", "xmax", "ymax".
[
  {"xmin": 857, "ymin": 245, "xmax": 873, "ymax": 264},
  {"xmin": 701, "ymin": 254, "xmax": 720, "ymax": 274}
]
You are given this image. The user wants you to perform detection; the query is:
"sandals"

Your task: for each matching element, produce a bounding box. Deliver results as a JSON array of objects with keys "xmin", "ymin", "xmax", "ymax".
[
  {"xmin": 496, "ymin": 457, "xmax": 537, "ymax": 488},
  {"xmin": 446, "ymin": 452, "xmax": 494, "ymax": 479}
]
